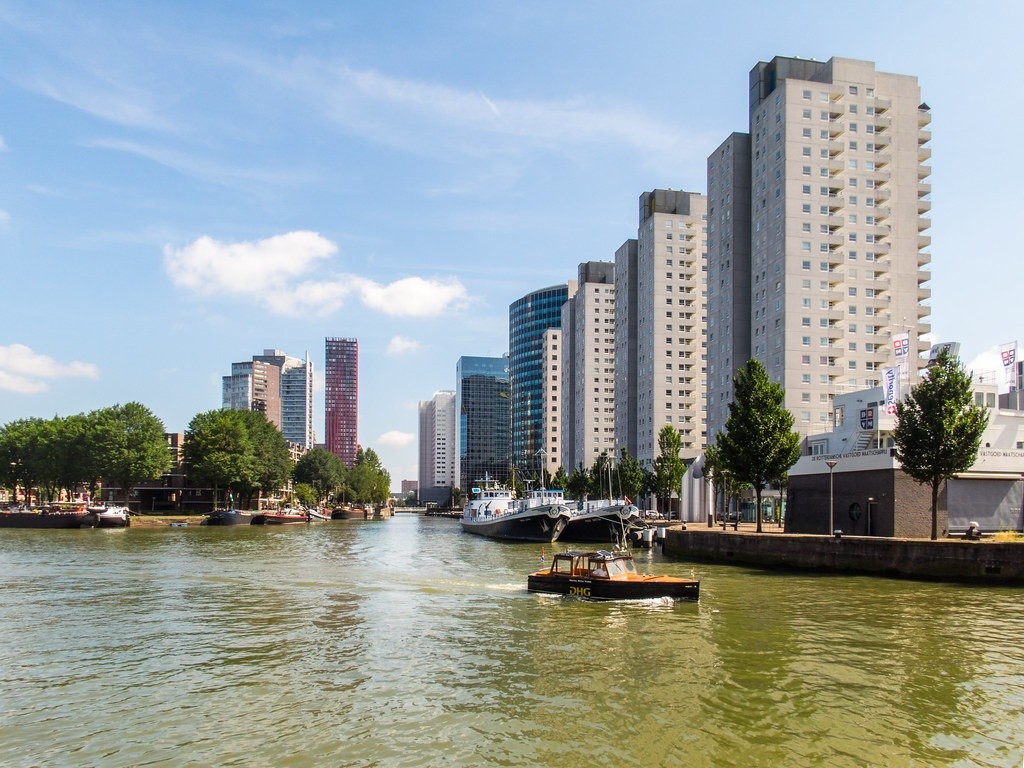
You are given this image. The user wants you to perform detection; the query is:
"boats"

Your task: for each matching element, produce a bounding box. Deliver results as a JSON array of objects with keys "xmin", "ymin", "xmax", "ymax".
[
  {"xmin": 0, "ymin": 499, "xmax": 91, "ymax": 528},
  {"xmin": 100, "ymin": 505, "xmax": 131, "ymax": 527},
  {"xmin": 460, "ymin": 448, "xmax": 642, "ymax": 541},
  {"xmin": 526, "ymin": 506, "xmax": 702, "ymax": 604},
  {"xmin": 328, "ymin": 505, "xmax": 376, "ymax": 522},
  {"xmin": 205, "ymin": 509, "xmax": 256, "ymax": 526},
  {"xmin": 249, "ymin": 509, "xmax": 313, "ymax": 527}
]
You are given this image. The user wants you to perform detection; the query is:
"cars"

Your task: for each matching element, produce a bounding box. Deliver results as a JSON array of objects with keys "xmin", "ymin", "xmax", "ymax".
[
  {"xmin": 643, "ymin": 509, "xmax": 664, "ymax": 520},
  {"xmin": 664, "ymin": 511, "xmax": 678, "ymax": 520}
]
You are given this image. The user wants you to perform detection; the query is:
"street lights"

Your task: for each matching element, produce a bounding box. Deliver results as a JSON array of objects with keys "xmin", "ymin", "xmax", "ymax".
[{"xmin": 826, "ymin": 461, "xmax": 839, "ymax": 536}]
